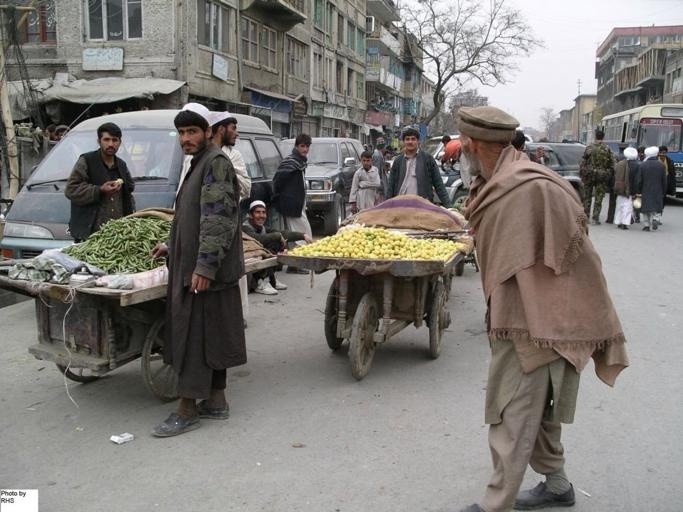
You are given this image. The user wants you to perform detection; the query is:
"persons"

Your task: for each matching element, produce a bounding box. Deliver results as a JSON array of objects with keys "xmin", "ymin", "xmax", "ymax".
[
  {"xmin": 345, "ymin": 127, "xmax": 454, "ymax": 214},
  {"xmin": 454, "ymin": 105, "xmax": 628, "ymax": 510},
  {"xmin": 270, "ymin": 132, "xmax": 312, "ymax": 275},
  {"xmin": 437, "ymin": 135, "xmax": 473, "ymax": 191},
  {"xmin": 240, "ymin": 201, "xmax": 312, "ymax": 297},
  {"xmin": 148, "ymin": 103, "xmax": 249, "ymax": 437},
  {"xmin": 64, "ymin": 123, "xmax": 137, "ymax": 244},
  {"xmin": 173, "ymin": 110, "xmax": 251, "ymax": 329},
  {"xmin": 510, "ymin": 130, "xmax": 545, "ymax": 164},
  {"xmin": 578, "ymin": 131, "xmax": 679, "ymax": 232}
]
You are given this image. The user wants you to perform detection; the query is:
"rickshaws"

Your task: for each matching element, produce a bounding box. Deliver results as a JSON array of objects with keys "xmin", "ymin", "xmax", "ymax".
[{"xmin": 0, "ymin": 249, "xmax": 278, "ymax": 406}]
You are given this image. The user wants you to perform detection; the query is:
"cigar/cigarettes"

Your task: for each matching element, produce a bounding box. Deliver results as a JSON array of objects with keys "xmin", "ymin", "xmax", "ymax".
[{"xmin": 194, "ymin": 287, "xmax": 197, "ymax": 291}]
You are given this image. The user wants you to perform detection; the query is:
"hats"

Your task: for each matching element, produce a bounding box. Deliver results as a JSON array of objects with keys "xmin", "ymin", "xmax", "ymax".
[
  {"xmin": 177, "ymin": 101, "xmax": 214, "ymax": 128},
  {"xmin": 455, "ymin": 103, "xmax": 520, "ymax": 144},
  {"xmin": 247, "ymin": 199, "xmax": 268, "ymax": 210},
  {"xmin": 206, "ymin": 109, "xmax": 237, "ymax": 128},
  {"xmin": 374, "ymin": 136, "xmax": 386, "ymax": 146}
]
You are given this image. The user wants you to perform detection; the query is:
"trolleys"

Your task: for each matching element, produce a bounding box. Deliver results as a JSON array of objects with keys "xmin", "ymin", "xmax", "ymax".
[{"xmin": 276, "ymin": 225, "xmax": 473, "ymax": 382}]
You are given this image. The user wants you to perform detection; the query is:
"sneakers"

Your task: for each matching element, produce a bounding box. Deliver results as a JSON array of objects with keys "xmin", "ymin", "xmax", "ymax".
[
  {"xmin": 629, "ymin": 214, "xmax": 641, "ymax": 225},
  {"xmin": 641, "ymin": 218, "xmax": 662, "ymax": 232},
  {"xmin": 271, "ymin": 271, "xmax": 288, "ymax": 291},
  {"xmin": 253, "ymin": 275, "xmax": 278, "ymax": 296},
  {"xmin": 616, "ymin": 223, "xmax": 629, "ymax": 231}
]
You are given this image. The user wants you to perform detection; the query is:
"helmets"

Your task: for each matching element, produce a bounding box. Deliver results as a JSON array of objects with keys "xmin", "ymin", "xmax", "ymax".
[{"xmin": 534, "ymin": 144, "xmax": 554, "ymax": 163}]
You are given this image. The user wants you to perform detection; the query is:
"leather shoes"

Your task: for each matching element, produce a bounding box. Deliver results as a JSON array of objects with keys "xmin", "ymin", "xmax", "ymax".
[
  {"xmin": 194, "ymin": 399, "xmax": 230, "ymax": 422},
  {"xmin": 510, "ymin": 480, "xmax": 577, "ymax": 511},
  {"xmin": 149, "ymin": 411, "xmax": 202, "ymax": 439},
  {"xmin": 457, "ymin": 502, "xmax": 494, "ymax": 512}
]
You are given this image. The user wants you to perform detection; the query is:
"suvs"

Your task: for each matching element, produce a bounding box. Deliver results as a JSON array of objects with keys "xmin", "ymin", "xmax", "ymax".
[
  {"xmin": 275, "ymin": 137, "xmax": 367, "ymax": 237},
  {"xmin": 385, "ymin": 133, "xmax": 589, "ymax": 217}
]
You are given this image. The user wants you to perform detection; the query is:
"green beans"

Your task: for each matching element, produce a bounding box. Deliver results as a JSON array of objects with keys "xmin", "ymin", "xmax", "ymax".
[{"xmin": 61, "ymin": 216, "xmax": 173, "ymax": 275}]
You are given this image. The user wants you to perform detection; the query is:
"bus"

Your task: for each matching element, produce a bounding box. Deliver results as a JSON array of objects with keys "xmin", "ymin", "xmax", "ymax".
[{"xmin": 599, "ymin": 102, "xmax": 683, "ymax": 198}]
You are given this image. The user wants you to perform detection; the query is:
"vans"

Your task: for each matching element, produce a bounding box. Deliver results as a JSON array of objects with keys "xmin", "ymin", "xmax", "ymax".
[{"xmin": 1, "ymin": 108, "xmax": 289, "ymax": 297}]
